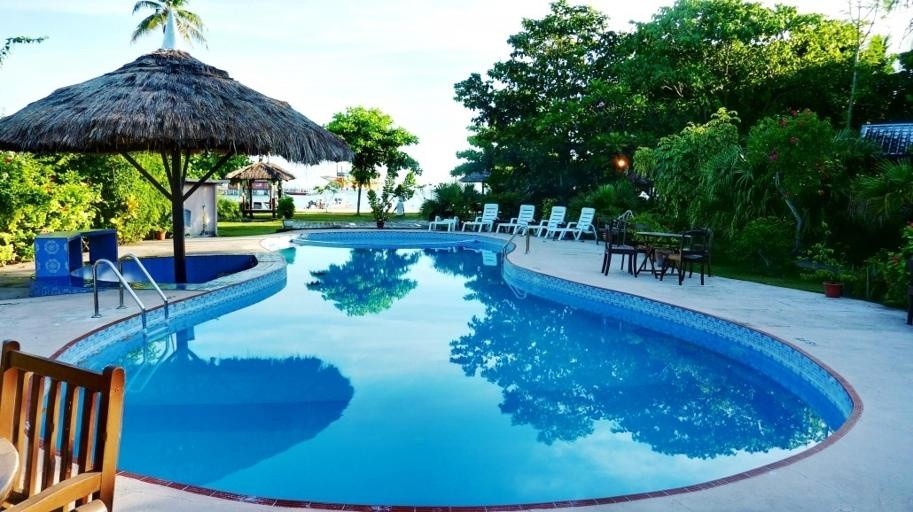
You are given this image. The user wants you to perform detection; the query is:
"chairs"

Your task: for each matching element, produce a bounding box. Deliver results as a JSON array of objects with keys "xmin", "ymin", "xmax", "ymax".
[
  {"xmin": 428, "ymin": 203, "xmax": 599, "ymax": 244},
  {"xmin": 601, "ymin": 217, "xmax": 712, "ymax": 285},
  {"xmin": 0, "ymin": 339, "xmax": 125, "ymax": 512}
]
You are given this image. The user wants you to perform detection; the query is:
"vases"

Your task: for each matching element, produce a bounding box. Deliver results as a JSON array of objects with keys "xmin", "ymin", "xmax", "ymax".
[{"xmin": 157, "ymin": 231, "xmax": 166, "ymax": 239}]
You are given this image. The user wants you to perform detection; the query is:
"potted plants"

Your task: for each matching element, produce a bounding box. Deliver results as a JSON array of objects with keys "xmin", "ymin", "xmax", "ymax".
[
  {"xmin": 799, "ymin": 267, "xmax": 859, "ymax": 297},
  {"xmin": 367, "ymin": 167, "xmax": 416, "ymax": 229}
]
